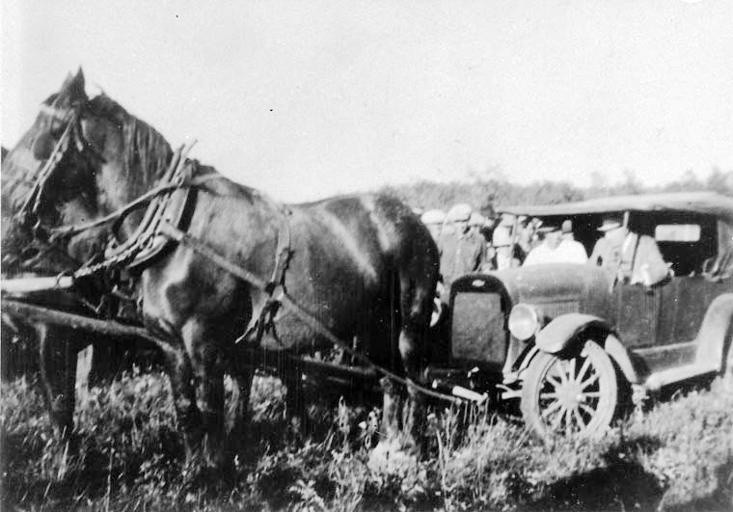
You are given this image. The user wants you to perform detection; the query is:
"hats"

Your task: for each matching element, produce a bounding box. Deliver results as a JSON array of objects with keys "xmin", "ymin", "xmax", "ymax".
[
  {"xmin": 537, "ymin": 220, "xmax": 559, "ymax": 233},
  {"xmin": 421, "ymin": 209, "xmax": 445, "ymax": 226},
  {"xmin": 596, "ymin": 216, "xmax": 622, "ymax": 231},
  {"xmin": 449, "ymin": 203, "xmax": 471, "ymax": 222}
]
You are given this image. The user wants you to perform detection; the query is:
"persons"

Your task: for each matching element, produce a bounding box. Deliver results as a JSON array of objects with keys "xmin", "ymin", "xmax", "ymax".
[{"xmin": 418, "ymin": 201, "xmax": 669, "ymax": 309}]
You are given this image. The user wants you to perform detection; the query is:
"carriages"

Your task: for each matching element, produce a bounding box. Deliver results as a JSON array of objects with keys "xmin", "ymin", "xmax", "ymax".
[{"xmin": 0, "ymin": 61, "xmax": 733, "ymax": 491}]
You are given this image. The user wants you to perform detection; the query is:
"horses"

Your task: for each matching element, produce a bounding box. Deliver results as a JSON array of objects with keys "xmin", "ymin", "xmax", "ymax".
[
  {"xmin": 1, "ymin": 66, "xmax": 443, "ymax": 495},
  {"xmin": 3, "ymin": 145, "xmax": 170, "ymax": 484}
]
